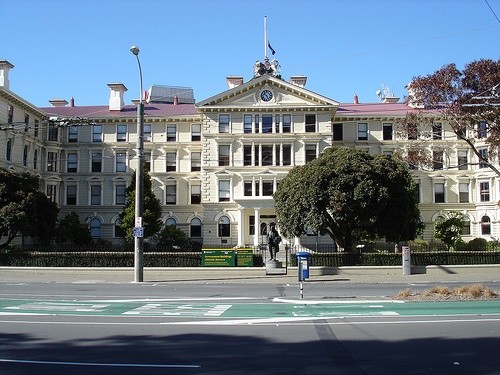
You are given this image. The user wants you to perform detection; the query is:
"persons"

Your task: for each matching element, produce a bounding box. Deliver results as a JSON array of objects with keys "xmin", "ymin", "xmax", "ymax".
[{"xmin": 265, "ymin": 226, "xmax": 281, "ymax": 261}]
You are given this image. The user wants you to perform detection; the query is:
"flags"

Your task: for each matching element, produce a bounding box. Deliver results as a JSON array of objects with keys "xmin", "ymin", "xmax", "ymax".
[{"xmin": 268, "ymin": 41, "xmax": 275, "ymax": 55}]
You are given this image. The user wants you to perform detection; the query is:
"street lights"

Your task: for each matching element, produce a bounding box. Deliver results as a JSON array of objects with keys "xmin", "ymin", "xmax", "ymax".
[{"xmin": 128, "ymin": 44, "xmax": 144, "ymax": 283}]
